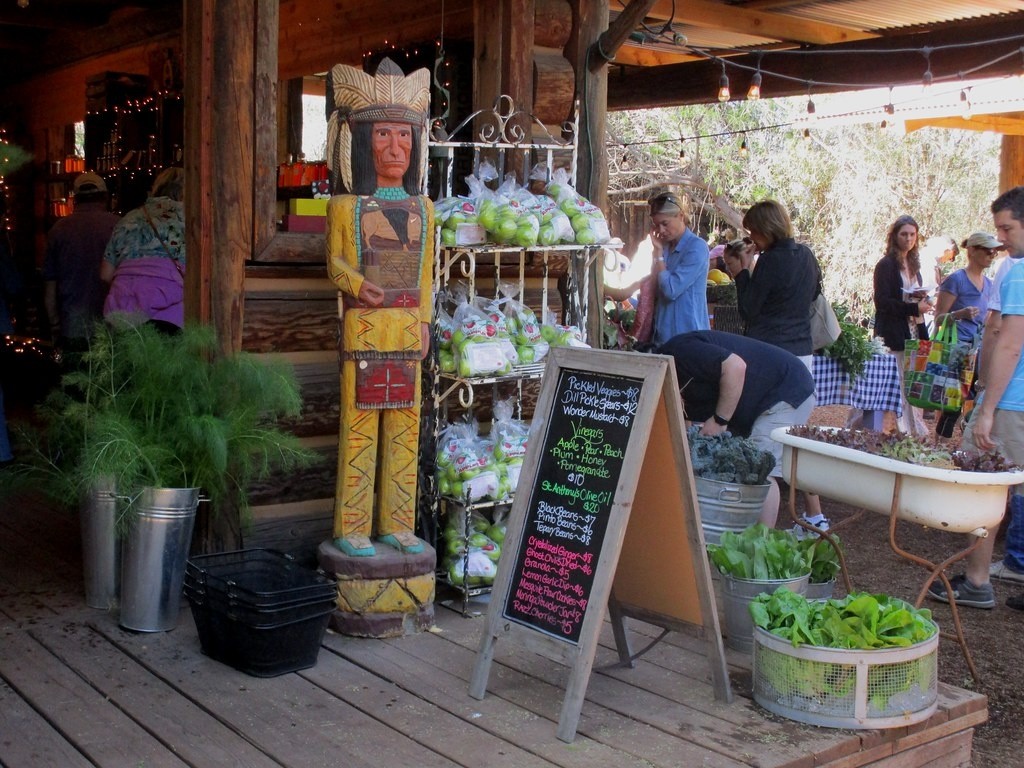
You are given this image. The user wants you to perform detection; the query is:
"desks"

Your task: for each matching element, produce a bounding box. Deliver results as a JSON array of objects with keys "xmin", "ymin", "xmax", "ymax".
[{"xmin": 810, "ymin": 352, "xmax": 903, "ymax": 432}]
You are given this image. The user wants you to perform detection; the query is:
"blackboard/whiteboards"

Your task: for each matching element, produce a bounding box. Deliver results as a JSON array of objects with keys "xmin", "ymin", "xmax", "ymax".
[{"xmin": 486, "ymin": 345, "xmax": 721, "ymax": 666}]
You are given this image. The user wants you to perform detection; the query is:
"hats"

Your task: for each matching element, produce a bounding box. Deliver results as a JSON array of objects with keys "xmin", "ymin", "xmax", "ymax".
[
  {"xmin": 967, "ymin": 232, "xmax": 1007, "ymax": 252},
  {"xmin": 74, "ymin": 173, "xmax": 107, "ymax": 195},
  {"xmin": 708, "ymin": 245, "xmax": 726, "ymax": 259}
]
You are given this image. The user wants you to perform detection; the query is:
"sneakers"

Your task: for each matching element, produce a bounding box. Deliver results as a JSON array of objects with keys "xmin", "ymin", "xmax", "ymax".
[
  {"xmin": 989, "ymin": 559, "xmax": 1024, "ymax": 586},
  {"xmin": 783, "ymin": 512, "xmax": 829, "ymax": 541},
  {"xmin": 927, "ymin": 571, "xmax": 995, "ymax": 609}
]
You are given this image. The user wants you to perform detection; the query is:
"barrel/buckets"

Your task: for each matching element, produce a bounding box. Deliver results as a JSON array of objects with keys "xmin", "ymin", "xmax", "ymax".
[
  {"xmin": 79, "ymin": 473, "xmax": 130, "ymax": 611},
  {"xmin": 806, "ymin": 576, "xmax": 837, "ymax": 601},
  {"xmin": 119, "ymin": 484, "xmax": 212, "ymax": 632},
  {"xmin": 716, "ymin": 565, "xmax": 811, "ymax": 655},
  {"xmin": 689, "ymin": 474, "xmax": 770, "ymax": 555}
]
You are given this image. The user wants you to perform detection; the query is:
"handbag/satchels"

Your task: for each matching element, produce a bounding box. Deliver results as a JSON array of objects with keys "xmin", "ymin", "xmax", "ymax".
[
  {"xmin": 800, "ymin": 243, "xmax": 841, "ymax": 350},
  {"xmin": 903, "ymin": 313, "xmax": 978, "ymax": 412},
  {"xmin": 630, "ymin": 250, "xmax": 657, "ymax": 344}
]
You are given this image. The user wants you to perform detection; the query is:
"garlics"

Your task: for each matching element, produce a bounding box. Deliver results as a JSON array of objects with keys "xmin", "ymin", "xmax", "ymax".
[{"xmin": 874, "ymin": 336, "xmax": 890, "ymax": 355}]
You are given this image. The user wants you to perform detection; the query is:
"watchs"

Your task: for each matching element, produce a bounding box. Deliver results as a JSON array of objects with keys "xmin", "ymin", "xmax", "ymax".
[
  {"xmin": 653, "ymin": 257, "xmax": 664, "ymax": 265},
  {"xmin": 714, "ymin": 412, "xmax": 729, "ymax": 426}
]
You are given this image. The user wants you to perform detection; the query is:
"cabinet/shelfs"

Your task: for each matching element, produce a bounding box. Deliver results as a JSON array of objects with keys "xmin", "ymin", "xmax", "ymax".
[{"xmin": 416, "ymin": 89, "xmax": 625, "ymax": 618}]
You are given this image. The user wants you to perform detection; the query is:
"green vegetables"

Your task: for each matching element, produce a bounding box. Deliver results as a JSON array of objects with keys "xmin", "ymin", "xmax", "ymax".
[
  {"xmin": 706, "ymin": 523, "xmax": 842, "ymax": 584},
  {"xmin": 684, "ymin": 424, "xmax": 776, "ymax": 486},
  {"xmin": 749, "ymin": 584, "xmax": 933, "ymax": 709},
  {"xmin": 816, "ymin": 300, "xmax": 877, "ymax": 390},
  {"xmin": 785, "ymin": 420, "xmax": 1024, "ymax": 473}
]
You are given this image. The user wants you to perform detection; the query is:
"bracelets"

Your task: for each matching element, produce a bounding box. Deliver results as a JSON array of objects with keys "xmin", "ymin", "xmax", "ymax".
[{"xmin": 950, "ymin": 311, "xmax": 961, "ymax": 322}]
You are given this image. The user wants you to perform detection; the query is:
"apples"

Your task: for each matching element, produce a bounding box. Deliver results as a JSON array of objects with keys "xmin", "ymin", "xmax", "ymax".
[
  {"xmin": 435, "ymin": 436, "xmax": 525, "ymax": 586},
  {"xmin": 435, "ymin": 308, "xmax": 576, "ymax": 377},
  {"xmin": 433, "ymin": 182, "xmax": 610, "ymax": 246}
]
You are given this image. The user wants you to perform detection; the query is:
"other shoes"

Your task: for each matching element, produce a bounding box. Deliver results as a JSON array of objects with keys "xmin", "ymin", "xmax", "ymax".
[
  {"xmin": 1004, "ymin": 593, "xmax": 1024, "ymax": 613},
  {"xmin": 922, "ymin": 408, "xmax": 936, "ymax": 420}
]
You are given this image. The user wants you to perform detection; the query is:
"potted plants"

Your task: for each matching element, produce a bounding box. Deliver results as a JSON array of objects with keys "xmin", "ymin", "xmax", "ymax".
[
  {"xmin": 771, "ymin": 421, "xmax": 1024, "ymax": 534},
  {"xmin": 1, "ymin": 308, "xmax": 328, "ymax": 634}
]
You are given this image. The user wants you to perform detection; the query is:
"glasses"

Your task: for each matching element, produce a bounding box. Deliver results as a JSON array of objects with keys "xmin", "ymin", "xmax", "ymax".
[
  {"xmin": 647, "ymin": 196, "xmax": 682, "ymax": 211},
  {"xmin": 976, "ymin": 244, "xmax": 998, "ymax": 258}
]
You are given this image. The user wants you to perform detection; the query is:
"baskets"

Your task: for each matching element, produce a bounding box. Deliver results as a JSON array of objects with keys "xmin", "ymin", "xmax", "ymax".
[{"xmin": 182, "ymin": 548, "xmax": 339, "ymax": 679}]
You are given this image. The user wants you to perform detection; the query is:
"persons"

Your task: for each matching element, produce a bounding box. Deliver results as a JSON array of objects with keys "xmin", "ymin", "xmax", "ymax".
[
  {"xmin": 874, "ymin": 216, "xmax": 1024, "ymax": 584},
  {"xmin": 606, "ymin": 238, "xmax": 631, "ymax": 272},
  {"xmin": 927, "ymin": 186, "xmax": 1024, "ymax": 608},
  {"xmin": 709, "ymin": 236, "xmax": 758, "ymax": 277},
  {"xmin": 325, "ymin": 56, "xmax": 436, "ymax": 558},
  {"xmin": 603, "ymin": 275, "xmax": 656, "ymax": 343},
  {"xmin": 632, "ymin": 330, "xmax": 815, "ymax": 532},
  {"xmin": 735, "ymin": 200, "xmax": 829, "ymax": 541},
  {"xmin": 47, "ymin": 173, "xmax": 121, "ymax": 396},
  {"xmin": 101, "ymin": 167, "xmax": 186, "ymax": 418},
  {"xmin": 649, "ymin": 192, "xmax": 710, "ymax": 352}
]
